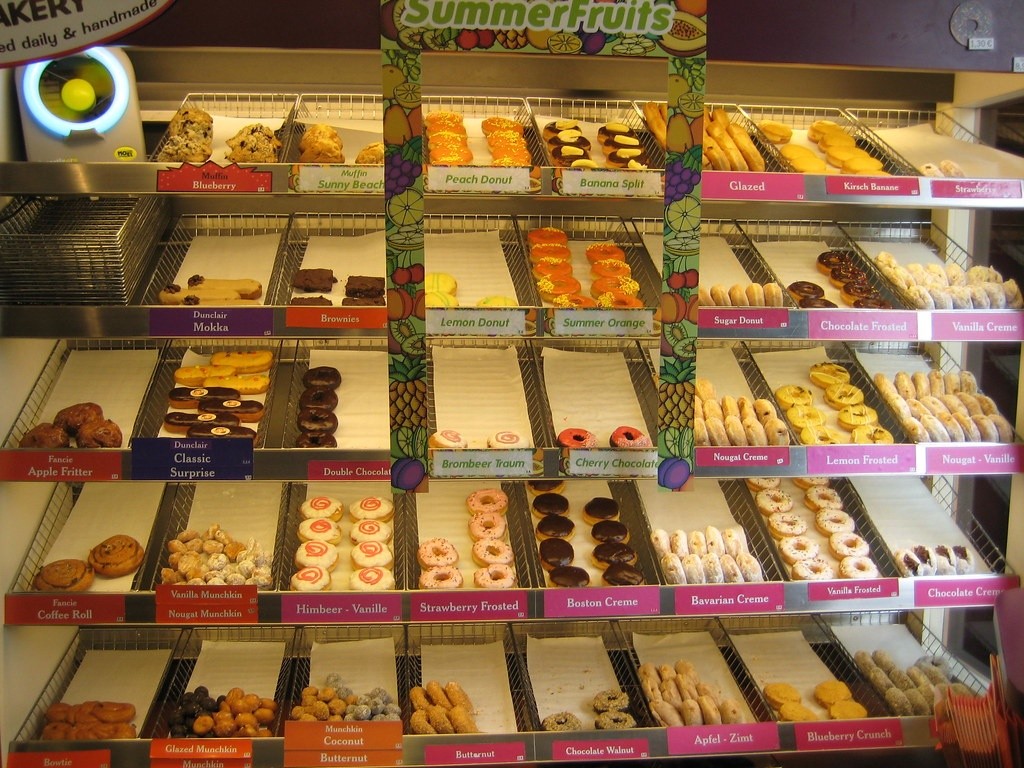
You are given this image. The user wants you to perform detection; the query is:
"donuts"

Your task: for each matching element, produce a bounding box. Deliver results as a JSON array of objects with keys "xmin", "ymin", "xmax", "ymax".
[
  {"xmin": 699, "ymin": 250, "xmax": 894, "ymax": 308},
  {"xmin": 543, "ymin": 661, "xmax": 745, "ymax": 731},
  {"xmin": 293, "ymin": 366, "xmax": 341, "ymax": 451},
  {"xmin": 689, "ymin": 362, "xmax": 1012, "ymax": 445},
  {"xmin": 746, "ymin": 477, "xmax": 879, "ymax": 581},
  {"xmin": 609, "ymin": 425, "xmax": 651, "ymax": 448},
  {"xmin": 416, "ymin": 487, "xmax": 516, "ymax": 589},
  {"xmin": 856, "ymin": 648, "xmax": 976, "ymax": 719},
  {"xmin": 557, "ymin": 428, "xmax": 597, "ymax": 448},
  {"xmin": 542, "ymin": 118, "xmax": 651, "ymax": 170},
  {"xmin": 526, "ymin": 226, "xmax": 647, "ymax": 307}
]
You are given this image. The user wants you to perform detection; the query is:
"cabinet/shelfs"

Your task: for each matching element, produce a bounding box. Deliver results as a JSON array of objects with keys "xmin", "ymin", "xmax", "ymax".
[{"xmin": 0, "ymin": 48, "xmax": 1024, "ymax": 768}]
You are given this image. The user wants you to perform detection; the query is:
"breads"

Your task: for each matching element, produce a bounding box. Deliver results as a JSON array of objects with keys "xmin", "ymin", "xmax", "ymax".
[
  {"xmin": 16, "ymin": 348, "xmax": 272, "ymax": 446},
  {"xmin": 43, "ymin": 674, "xmax": 399, "ymax": 741},
  {"xmin": 428, "ymin": 430, "xmax": 468, "ymax": 449},
  {"xmin": 30, "ymin": 496, "xmax": 397, "ymax": 591},
  {"xmin": 154, "ymin": 106, "xmax": 532, "ymax": 165},
  {"xmin": 644, "ymin": 101, "xmax": 965, "ymax": 177},
  {"xmin": 487, "ymin": 430, "xmax": 531, "ymax": 448},
  {"xmin": 424, "ymin": 272, "xmax": 520, "ymax": 307},
  {"xmin": 874, "ymin": 251, "xmax": 1023, "ymax": 309},
  {"xmin": 763, "ymin": 681, "xmax": 866, "ymax": 723},
  {"xmin": 159, "ymin": 268, "xmax": 388, "ymax": 307},
  {"xmin": 529, "ymin": 479, "xmax": 764, "ymax": 586},
  {"xmin": 895, "ymin": 544, "xmax": 974, "ymax": 576}
]
